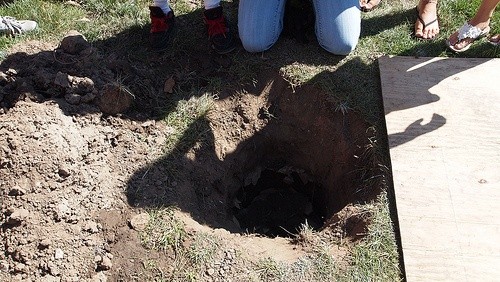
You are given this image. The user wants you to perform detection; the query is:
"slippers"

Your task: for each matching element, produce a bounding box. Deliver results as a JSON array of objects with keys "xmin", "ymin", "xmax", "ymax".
[
  {"xmin": 413, "ymin": 3, "xmax": 441, "ymax": 43},
  {"xmin": 442, "ymin": 19, "xmax": 493, "ymax": 54}
]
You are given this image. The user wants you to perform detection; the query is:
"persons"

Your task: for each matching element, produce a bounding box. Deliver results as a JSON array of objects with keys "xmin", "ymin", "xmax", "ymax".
[
  {"xmin": 147, "ymin": 0, "xmax": 239, "ymax": 55},
  {"xmin": 447, "ymin": 0, "xmax": 500, "ymax": 54},
  {"xmin": 359, "ymin": 0, "xmax": 441, "ymax": 41},
  {"xmin": 237, "ymin": 0, "xmax": 363, "ymax": 56}
]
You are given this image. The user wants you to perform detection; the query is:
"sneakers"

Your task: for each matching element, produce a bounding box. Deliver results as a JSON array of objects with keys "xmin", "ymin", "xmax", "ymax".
[
  {"xmin": 147, "ymin": 3, "xmax": 177, "ymax": 53},
  {"xmin": 202, "ymin": 4, "xmax": 241, "ymax": 57}
]
